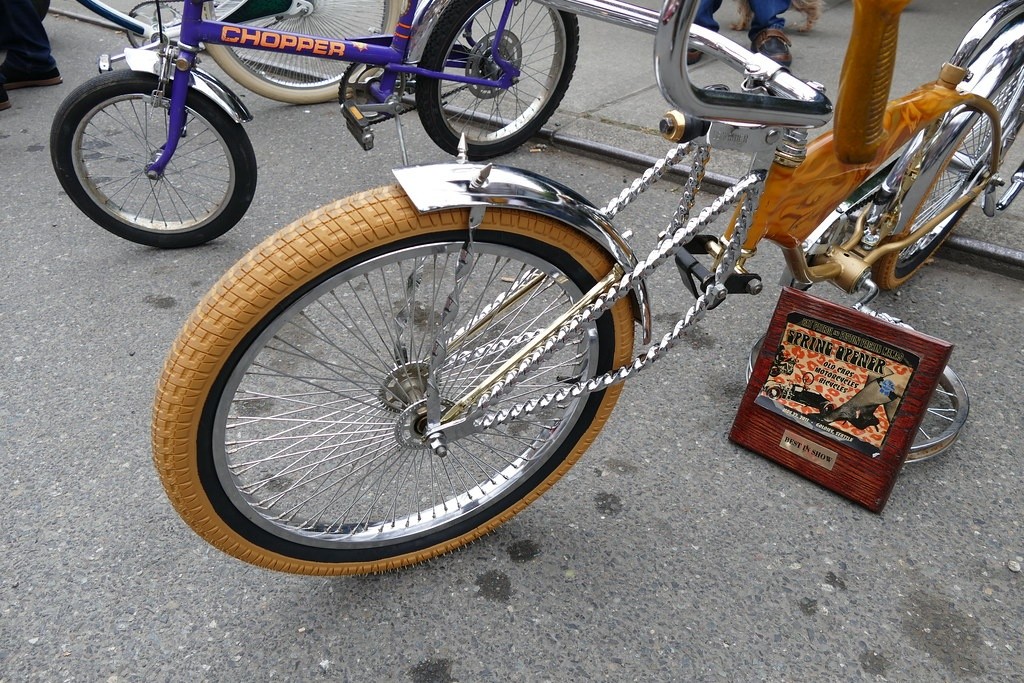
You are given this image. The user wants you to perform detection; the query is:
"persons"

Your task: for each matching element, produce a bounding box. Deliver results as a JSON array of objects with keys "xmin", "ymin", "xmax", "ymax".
[
  {"xmin": 687, "ymin": 0, "xmax": 792, "ymax": 66},
  {"xmin": 0, "ymin": 0, "xmax": 64, "ymax": 110}
]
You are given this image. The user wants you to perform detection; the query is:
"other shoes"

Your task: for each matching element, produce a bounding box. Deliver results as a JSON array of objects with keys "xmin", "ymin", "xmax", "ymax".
[
  {"xmin": 0, "ymin": 61, "xmax": 63, "ymax": 90},
  {"xmin": 0, "ymin": 85, "xmax": 12, "ymax": 112}
]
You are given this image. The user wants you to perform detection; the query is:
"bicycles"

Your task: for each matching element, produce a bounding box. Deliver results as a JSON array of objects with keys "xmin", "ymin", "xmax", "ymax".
[
  {"xmin": 150, "ymin": 0, "xmax": 1024, "ymax": 576},
  {"xmin": 49, "ymin": 0, "xmax": 579, "ymax": 251}
]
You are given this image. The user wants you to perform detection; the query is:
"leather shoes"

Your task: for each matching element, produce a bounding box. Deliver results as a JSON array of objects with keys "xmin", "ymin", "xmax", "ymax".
[
  {"xmin": 687, "ymin": 48, "xmax": 704, "ymax": 65},
  {"xmin": 751, "ymin": 30, "xmax": 792, "ymax": 67}
]
